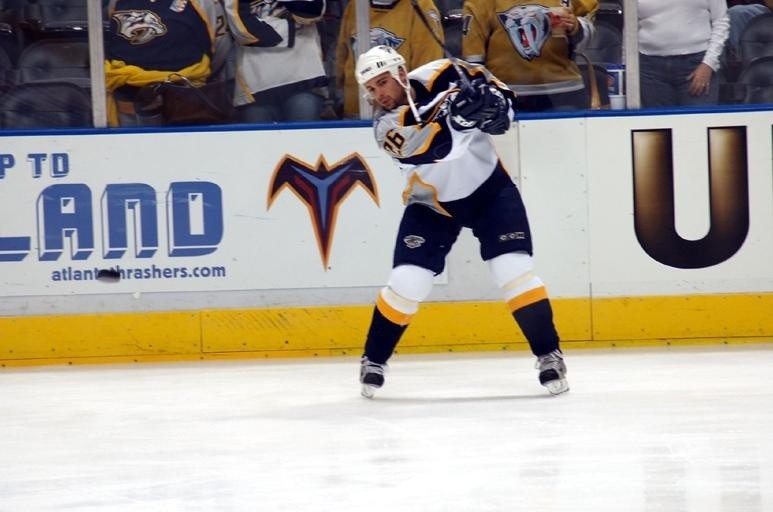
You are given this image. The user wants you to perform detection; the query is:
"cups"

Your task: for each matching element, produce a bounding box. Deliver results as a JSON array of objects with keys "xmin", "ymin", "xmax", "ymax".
[
  {"xmin": 548, "ymin": 7, "xmax": 568, "ymax": 38},
  {"xmin": 605, "ymin": 63, "xmax": 627, "ymax": 110}
]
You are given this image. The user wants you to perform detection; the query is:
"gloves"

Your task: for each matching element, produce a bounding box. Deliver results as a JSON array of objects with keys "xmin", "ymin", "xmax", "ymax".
[{"xmin": 449, "ymin": 79, "xmax": 512, "ymax": 136}]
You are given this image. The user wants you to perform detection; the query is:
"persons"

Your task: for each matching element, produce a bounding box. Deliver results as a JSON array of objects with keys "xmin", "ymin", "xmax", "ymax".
[
  {"xmin": 102, "ymin": 0, "xmax": 773, "ymax": 126},
  {"xmin": 355, "ymin": 46, "xmax": 569, "ymax": 388}
]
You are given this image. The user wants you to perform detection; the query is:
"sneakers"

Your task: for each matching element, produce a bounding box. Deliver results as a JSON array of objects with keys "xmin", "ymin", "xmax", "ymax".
[
  {"xmin": 537, "ymin": 347, "xmax": 570, "ymax": 384},
  {"xmin": 358, "ymin": 353, "xmax": 385, "ymax": 390}
]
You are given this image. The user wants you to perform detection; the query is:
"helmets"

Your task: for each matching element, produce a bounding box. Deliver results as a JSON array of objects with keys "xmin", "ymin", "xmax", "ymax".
[{"xmin": 354, "ymin": 45, "xmax": 410, "ymax": 94}]
